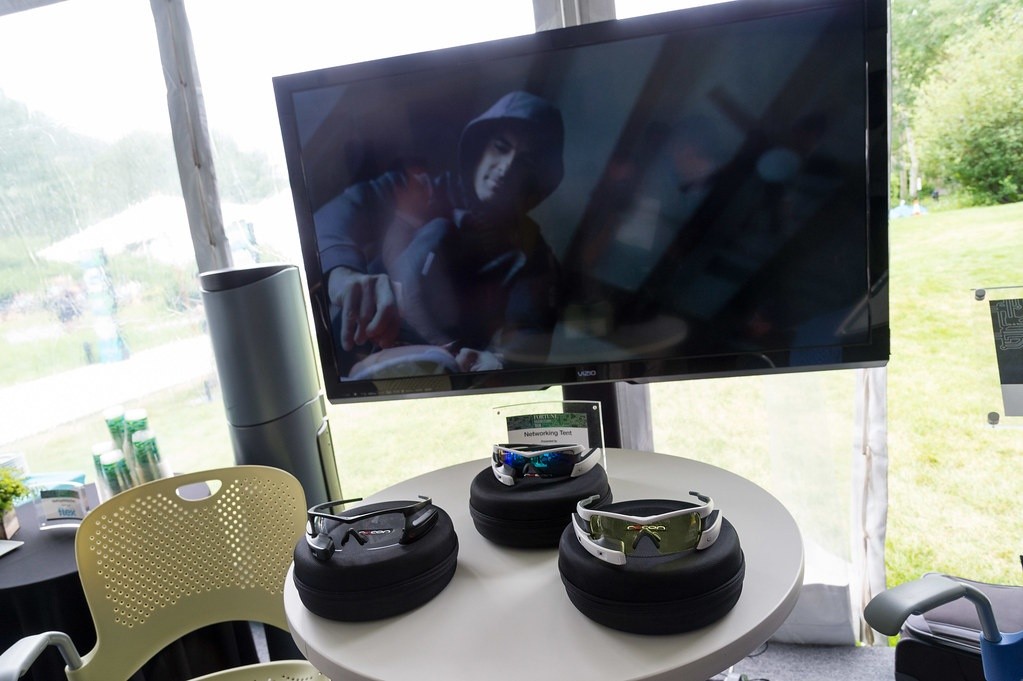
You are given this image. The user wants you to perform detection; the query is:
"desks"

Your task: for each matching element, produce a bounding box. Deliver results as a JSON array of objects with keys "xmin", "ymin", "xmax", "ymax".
[
  {"xmin": 283, "ymin": 438, "xmax": 805, "ymax": 681},
  {"xmin": 0, "ymin": 461, "xmax": 260, "ymax": 681},
  {"xmin": 490, "ymin": 316, "xmax": 687, "ymax": 365}
]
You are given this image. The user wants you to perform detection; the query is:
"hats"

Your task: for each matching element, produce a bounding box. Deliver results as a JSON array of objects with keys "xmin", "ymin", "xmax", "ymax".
[{"xmin": 458, "ymin": 92, "xmax": 567, "ymax": 215}]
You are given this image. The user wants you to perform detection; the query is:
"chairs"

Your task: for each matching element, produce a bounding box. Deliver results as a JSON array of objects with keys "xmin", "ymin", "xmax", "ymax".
[{"xmin": 0, "ymin": 464, "xmax": 336, "ymax": 681}]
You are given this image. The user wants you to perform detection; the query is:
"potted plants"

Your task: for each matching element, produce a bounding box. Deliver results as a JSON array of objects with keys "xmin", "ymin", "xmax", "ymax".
[{"xmin": 0, "ymin": 467, "xmax": 35, "ymax": 540}]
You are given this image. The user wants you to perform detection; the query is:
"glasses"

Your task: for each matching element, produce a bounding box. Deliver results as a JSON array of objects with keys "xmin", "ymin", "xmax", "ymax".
[
  {"xmin": 490, "ymin": 443, "xmax": 599, "ymax": 488},
  {"xmin": 304, "ymin": 493, "xmax": 439, "ymax": 564},
  {"xmin": 572, "ymin": 491, "xmax": 723, "ymax": 565}
]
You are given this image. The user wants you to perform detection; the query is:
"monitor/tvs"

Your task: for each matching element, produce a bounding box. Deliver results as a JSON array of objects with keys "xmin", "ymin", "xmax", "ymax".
[{"xmin": 271, "ymin": 0, "xmax": 890, "ymax": 405}]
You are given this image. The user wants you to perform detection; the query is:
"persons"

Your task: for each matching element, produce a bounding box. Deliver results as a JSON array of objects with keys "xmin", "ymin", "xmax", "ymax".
[{"xmin": 313, "ymin": 91, "xmax": 564, "ymax": 372}]
